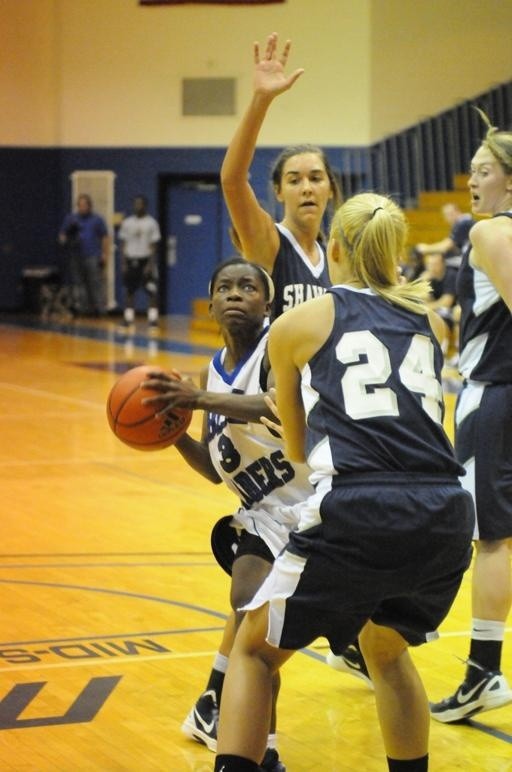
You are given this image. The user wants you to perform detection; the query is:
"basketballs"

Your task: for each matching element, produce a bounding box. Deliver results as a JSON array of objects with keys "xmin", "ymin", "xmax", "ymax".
[{"xmin": 105, "ymin": 364, "xmax": 192, "ymax": 452}]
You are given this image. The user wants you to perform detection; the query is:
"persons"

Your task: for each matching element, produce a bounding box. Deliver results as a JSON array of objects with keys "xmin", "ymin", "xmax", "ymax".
[
  {"xmin": 215, "ymin": 195, "xmax": 475, "ymax": 772},
  {"xmin": 138, "ymin": 255, "xmax": 383, "ymax": 759},
  {"xmin": 117, "ymin": 194, "xmax": 165, "ymax": 332},
  {"xmin": 57, "ymin": 193, "xmax": 110, "ymax": 316},
  {"xmin": 429, "ymin": 110, "xmax": 512, "ymax": 722},
  {"xmin": 397, "ymin": 205, "xmax": 474, "ymax": 368},
  {"xmin": 221, "ymin": 32, "xmax": 345, "ymax": 324}
]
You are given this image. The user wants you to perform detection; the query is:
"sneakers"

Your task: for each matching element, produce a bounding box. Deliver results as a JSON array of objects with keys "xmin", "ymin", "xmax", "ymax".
[
  {"xmin": 118, "ymin": 320, "xmax": 160, "ymax": 331},
  {"xmin": 427, "ymin": 669, "xmax": 512, "ymax": 724},
  {"xmin": 180, "ymin": 699, "xmax": 219, "ymax": 753},
  {"xmin": 325, "ymin": 643, "xmax": 379, "ymax": 690}
]
